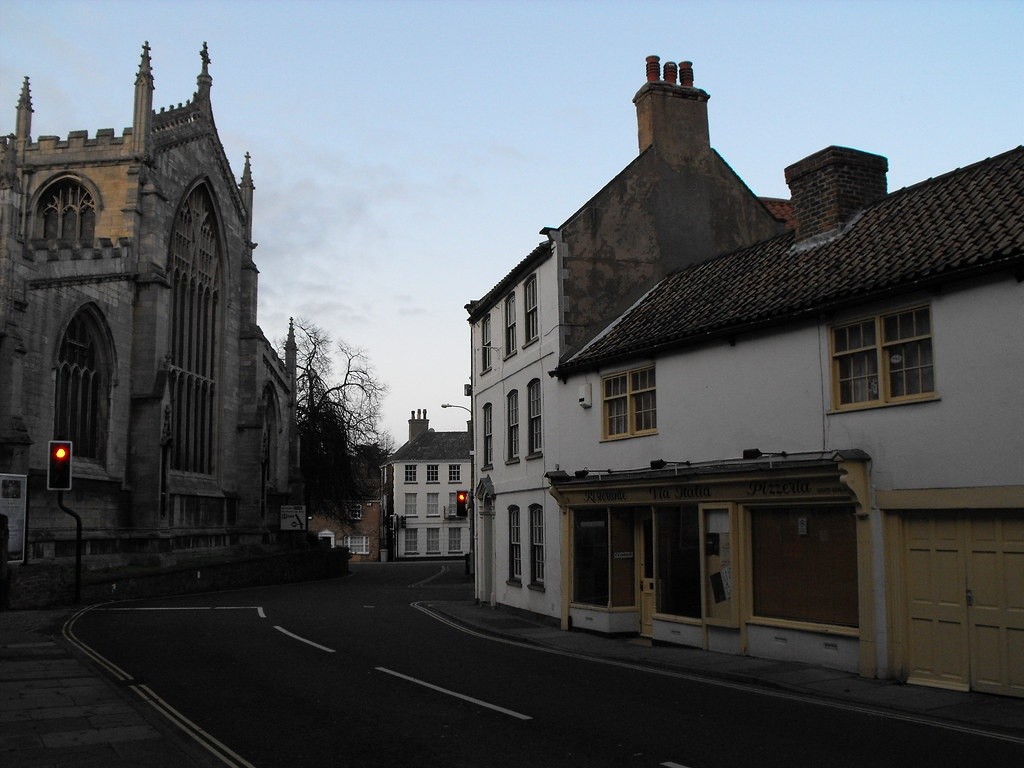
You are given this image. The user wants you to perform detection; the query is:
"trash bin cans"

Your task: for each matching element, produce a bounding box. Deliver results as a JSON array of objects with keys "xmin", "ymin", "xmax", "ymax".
[
  {"xmin": 379, "ymin": 549, "xmax": 388, "ymax": 562},
  {"xmin": 464, "ymin": 554, "xmax": 470, "ymax": 574}
]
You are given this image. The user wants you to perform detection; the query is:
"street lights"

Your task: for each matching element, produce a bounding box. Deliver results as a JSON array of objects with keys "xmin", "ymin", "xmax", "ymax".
[{"xmin": 441, "ymin": 403, "xmax": 474, "ymax": 577}]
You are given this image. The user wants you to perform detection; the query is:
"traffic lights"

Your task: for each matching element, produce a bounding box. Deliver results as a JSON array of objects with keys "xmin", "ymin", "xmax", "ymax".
[
  {"xmin": 457, "ymin": 491, "xmax": 467, "ymax": 516},
  {"xmin": 47, "ymin": 441, "xmax": 73, "ymax": 491}
]
